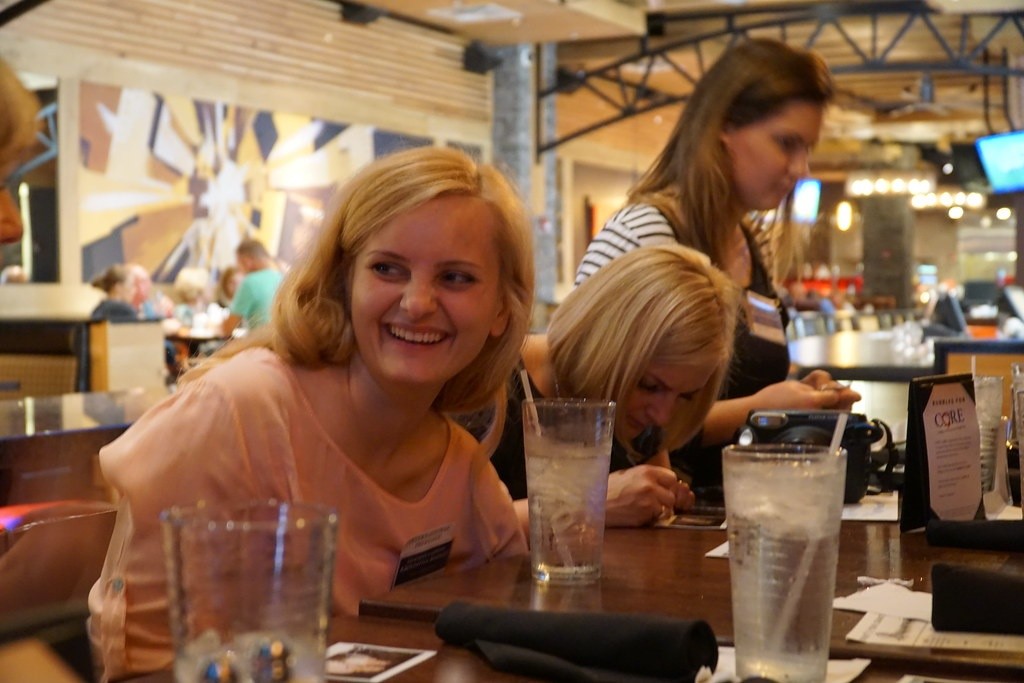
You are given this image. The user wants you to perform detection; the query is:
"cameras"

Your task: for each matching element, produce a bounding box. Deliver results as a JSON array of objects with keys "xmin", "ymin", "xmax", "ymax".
[{"xmin": 734, "ymin": 409, "xmax": 870, "ymax": 503}]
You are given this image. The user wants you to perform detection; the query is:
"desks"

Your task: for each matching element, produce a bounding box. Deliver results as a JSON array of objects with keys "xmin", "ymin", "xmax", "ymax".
[
  {"xmin": 0, "ymin": 390, "xmax": 167, "ymax": 507},
  {"xmin": 133, "ymin": 516, "xmax": 1024, "ymax": 683}
]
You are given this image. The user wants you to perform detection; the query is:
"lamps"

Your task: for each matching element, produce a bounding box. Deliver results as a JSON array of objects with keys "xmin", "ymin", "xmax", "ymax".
[
  {"xmin": 558, "ymin": 67, "xmax": 582, "ymax": 91},
  {"xmin": 464, "ymin": 37, "xmax": 503, "ymax": 73},
  {"xmin": 334, "ymin": 1, "xmax": 384, "ymax": 25}
]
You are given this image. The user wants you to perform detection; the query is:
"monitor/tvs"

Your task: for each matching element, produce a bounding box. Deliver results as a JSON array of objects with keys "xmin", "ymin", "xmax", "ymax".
[
  {"xmin": 793, "ymin": 177, "xmax": 823, "ymax": 225},
  {"xmin": 978, "ymin": 130, "xmax": 1024, "ymax": 193}
]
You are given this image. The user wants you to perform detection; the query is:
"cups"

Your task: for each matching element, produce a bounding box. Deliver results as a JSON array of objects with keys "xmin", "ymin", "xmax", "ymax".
[
  {"xmin": 160, "ymin": 502, "xmax": 335, "ymax": 683},
  {"xmin": 724, "ymin": 441, "xmax": 847, "ymax": 680},
  {"xmin": 973, "ymin": 376, "xmax": 1004, "ymax": 495},
  {"xmin": 521, "ymin": 399, "xmax": 618, "ymax": 587}
]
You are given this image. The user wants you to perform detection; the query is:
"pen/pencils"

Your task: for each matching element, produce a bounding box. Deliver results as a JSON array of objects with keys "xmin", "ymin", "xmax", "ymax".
[{"xmin": 627, "ymin": 453, "xmax": 638, "ymax": 468}]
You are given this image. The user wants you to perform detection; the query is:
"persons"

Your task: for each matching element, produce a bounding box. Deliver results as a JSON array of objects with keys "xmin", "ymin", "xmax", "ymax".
[
  {"xmin": 84, "ymin": 142, "xmax": 536, "ymax": 683},
  {"xmin": 574, "ymin": 39, "xmax": 861, "ymax": 486},
  {"xmin": 449, "ymin": 243, "xmax": 733, "ymax": 548}
]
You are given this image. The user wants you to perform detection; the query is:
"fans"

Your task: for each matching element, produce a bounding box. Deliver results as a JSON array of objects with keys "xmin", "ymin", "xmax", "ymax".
[{"xmin": 862, "ymin": 72, "xmax": 993, "ymax": 115}]
